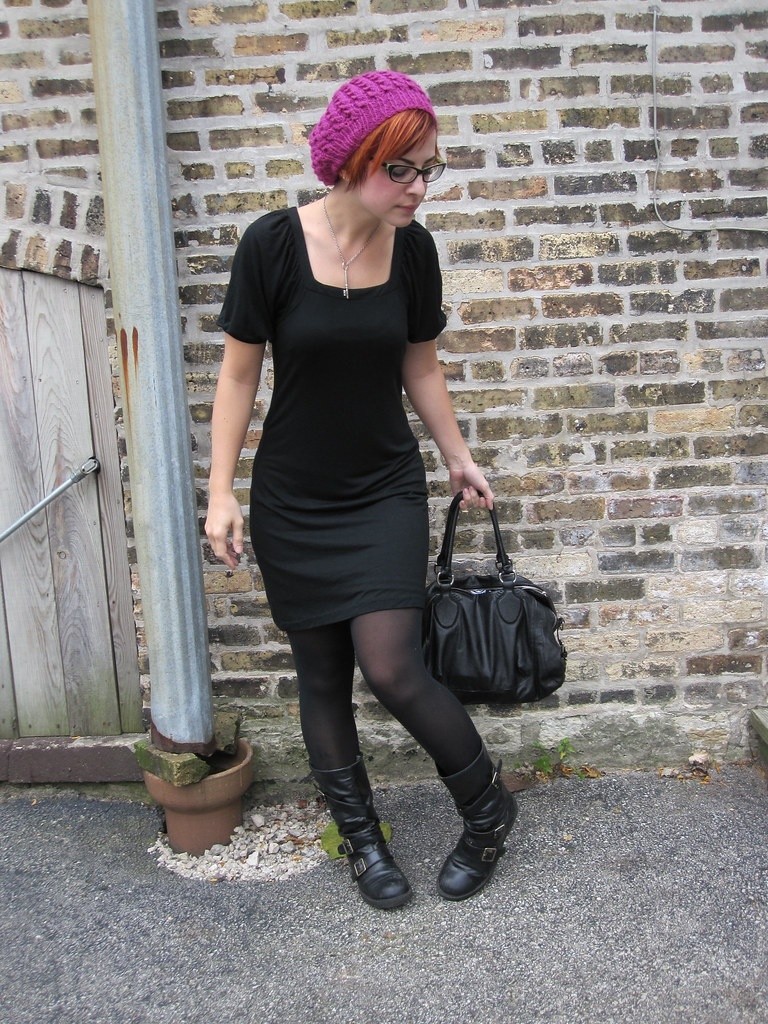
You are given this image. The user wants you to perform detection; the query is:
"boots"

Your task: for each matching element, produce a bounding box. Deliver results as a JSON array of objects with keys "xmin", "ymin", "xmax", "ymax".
[
  {"xmin": 436, "ymin": 739, "xmax": 518, "ymax": 899},
  {"xmin": 308, "ymin": 752, "xmax": 411, "ymax": 907}
]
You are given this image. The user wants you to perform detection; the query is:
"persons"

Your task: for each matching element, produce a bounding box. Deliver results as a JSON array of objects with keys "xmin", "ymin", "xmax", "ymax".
[{"xmin": 204, "ymin": 69, "xmax": 519, "ymax": 909}]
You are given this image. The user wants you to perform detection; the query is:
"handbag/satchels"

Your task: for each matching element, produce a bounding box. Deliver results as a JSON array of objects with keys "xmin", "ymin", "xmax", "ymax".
[{"xmin": 421, "ymin": 487, "xmax": 566, "ymax": 704}]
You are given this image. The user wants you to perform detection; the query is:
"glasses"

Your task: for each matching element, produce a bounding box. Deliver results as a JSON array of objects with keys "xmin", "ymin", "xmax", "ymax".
[{"xmin": 370, "ymin": 154, "xmax": 446, "ymax": 184}]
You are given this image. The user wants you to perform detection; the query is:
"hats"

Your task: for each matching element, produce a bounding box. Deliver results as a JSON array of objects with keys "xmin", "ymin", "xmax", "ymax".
[{"xmin": 308, "ymin": 69, "xmax": 435, "ymax": 187}]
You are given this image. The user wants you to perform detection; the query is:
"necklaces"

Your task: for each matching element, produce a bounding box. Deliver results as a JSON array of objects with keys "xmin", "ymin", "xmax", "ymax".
[{"xmin": 324, "ymin": 194, "xmax": 382, "ymax": 301}]
